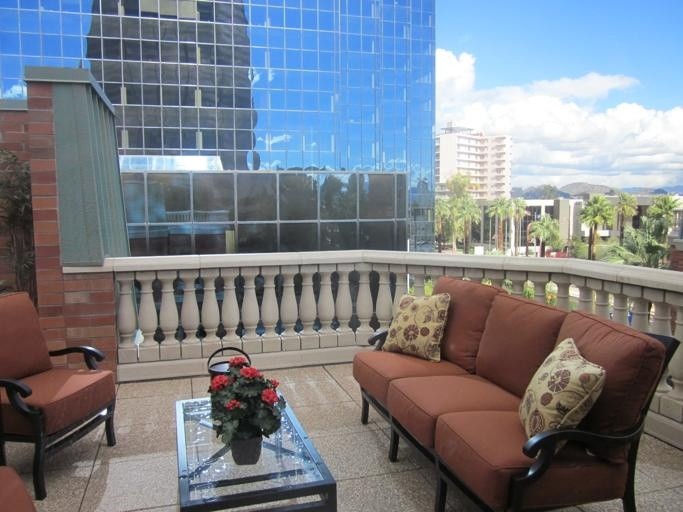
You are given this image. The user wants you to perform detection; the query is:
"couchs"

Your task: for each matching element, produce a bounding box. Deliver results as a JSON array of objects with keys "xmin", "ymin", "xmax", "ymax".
[{"xmin": 353, "ymin": 274, "xmax": 680, "ymax": 512}]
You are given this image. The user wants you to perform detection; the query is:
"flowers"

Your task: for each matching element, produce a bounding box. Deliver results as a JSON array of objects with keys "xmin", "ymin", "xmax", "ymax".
[{"xmin": 207, "ymin": 353, "xmax": 288, "ymax": 444}]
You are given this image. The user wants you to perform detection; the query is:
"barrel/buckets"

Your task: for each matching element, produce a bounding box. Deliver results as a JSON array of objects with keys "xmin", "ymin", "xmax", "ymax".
[{"xmin": 206, "ymin": 348, "xmax": 252, "ymax": 417}]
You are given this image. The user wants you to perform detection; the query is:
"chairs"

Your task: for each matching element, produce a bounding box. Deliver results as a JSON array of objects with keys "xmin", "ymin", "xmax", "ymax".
[{"xmin": 0, "ymin": 290, "xmax": 119, "ymax": 501}]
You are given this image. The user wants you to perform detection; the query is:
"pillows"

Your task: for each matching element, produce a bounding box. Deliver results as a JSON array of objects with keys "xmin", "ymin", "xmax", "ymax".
[
  {"xmin": 380, "ymin": 290, "xmax": 451, "ymax": 362},
  {"xmin": 517, "ymin": 337, "xmax": 607, "ymax": 459}
]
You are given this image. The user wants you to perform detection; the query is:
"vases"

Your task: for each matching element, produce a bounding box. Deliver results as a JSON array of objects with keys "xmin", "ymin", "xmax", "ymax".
[{"xmin": 230, "ymin": 436, "xmax": 261, "ymax": 466}]
